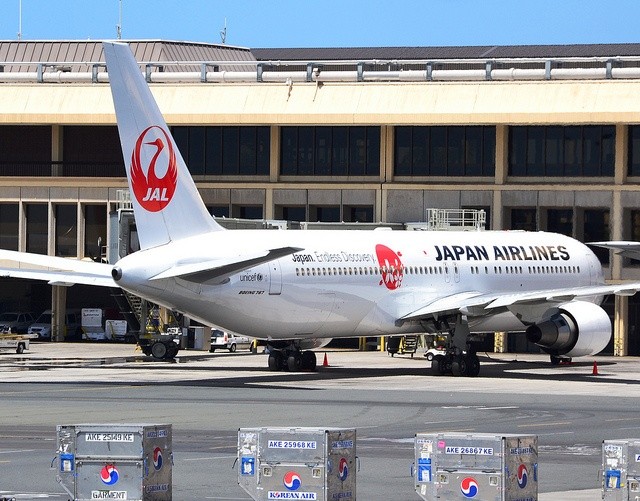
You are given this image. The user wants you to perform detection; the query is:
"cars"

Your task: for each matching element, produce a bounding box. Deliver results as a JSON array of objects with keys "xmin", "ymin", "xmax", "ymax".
[{"xmin": 423, "ymin": 346, "xmax": 448, "ymax": 360}]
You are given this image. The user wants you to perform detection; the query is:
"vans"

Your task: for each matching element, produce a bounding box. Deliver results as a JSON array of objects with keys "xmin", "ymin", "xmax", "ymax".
[
  {"xmin": 210, "ymin": 325, "xmax": 258, "ymax": 353},
  {"xmin": 29, "ymin": 308, "xmax": 81, "ymax": 339}
]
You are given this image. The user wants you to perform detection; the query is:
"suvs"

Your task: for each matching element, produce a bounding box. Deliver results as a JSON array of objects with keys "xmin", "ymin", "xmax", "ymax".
[{"xmin": 0, "ymin": 311, "xmax": 37, "ymax": 333}]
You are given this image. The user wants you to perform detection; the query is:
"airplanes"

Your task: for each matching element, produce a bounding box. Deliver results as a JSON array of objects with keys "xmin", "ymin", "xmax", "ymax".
[{"xmin": 0, "ymin": 39, "xmax": 640, "ymax": 376}]
